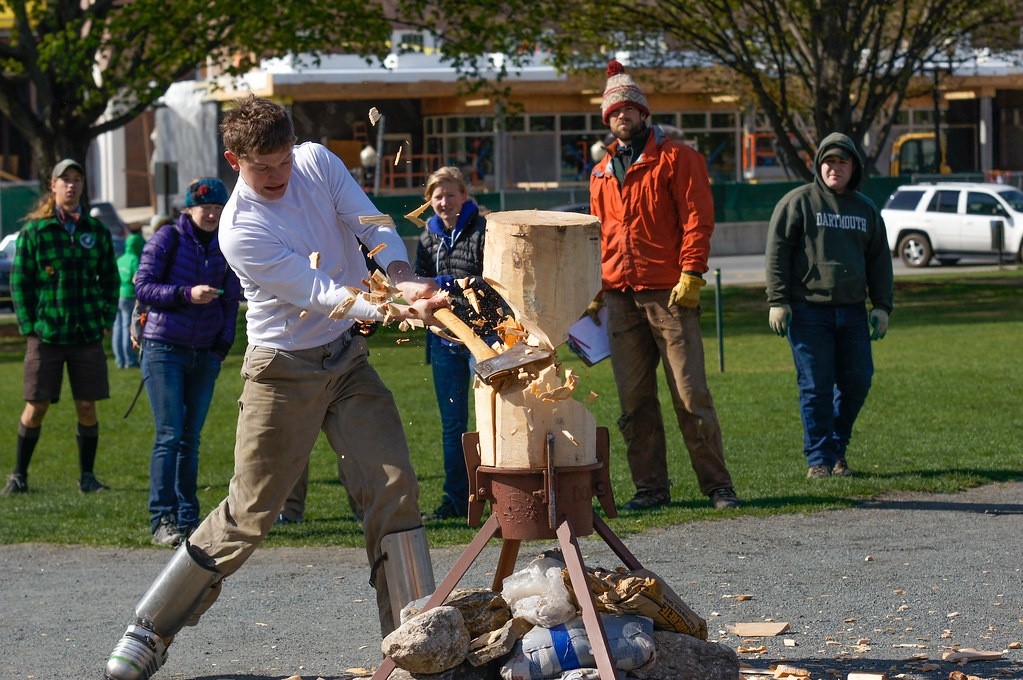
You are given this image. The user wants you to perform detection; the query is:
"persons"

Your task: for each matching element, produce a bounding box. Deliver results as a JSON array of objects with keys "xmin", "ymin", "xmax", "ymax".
[
  {"xmin": 578, "ymin": 59, "xmax": 739, "ymax": 513},
  {"xmin": 276, "ymin": 236, "xmax": 385, "ymax": 525},
  {"xmin": 104, "ymin": 100, "xmax": 452, "ymax": 680},
  {"xmin": 765, "ymin": 133, "xmax": 893, "ymax": 477},
  {"xmin": 110, "ymin": 214, "xmax": 175, "ymax": 368},
  {"xmin": 132, "ymin": 178, "xmax": 240, "ymax": 545},
  {"xmin": 0, "ymin": 158, "xmax": 120, "ymax": 496},
  {"xmin": 414, "ymin": 166, "xmax": 486, "ymax": 519}
]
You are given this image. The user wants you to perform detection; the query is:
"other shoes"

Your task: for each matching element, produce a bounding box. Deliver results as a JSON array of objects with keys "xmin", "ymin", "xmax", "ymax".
[
  {"xmin": 806, "ymin": 456, "xmax": 855, "ymax": 478},
  {"xmin": 421, "ymin": 495, "xmax": 469, "ymax": 521},
  {"xmin": 80, "ymin": 472, "xmax": 110, "ymax": 494},
  {"xmin": 1, "ymin": 472, "xmax": 28, "ymax": 496}
]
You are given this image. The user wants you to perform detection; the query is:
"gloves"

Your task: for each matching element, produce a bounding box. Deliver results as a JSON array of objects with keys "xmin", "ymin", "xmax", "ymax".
[
  {"xmin": 667, "ymin": 272, "xmax": 707, "ymax": 310},
  {"xmin": 580, "ymin": 301, "xmax": 605, "ymax": 326},
  {"xmin": 869, "ymin": 307, "xmax": 889, "ymax": 341},
  {"xmin": 769, "ymin": 304, "xmax": 792, "ymax": 338}
]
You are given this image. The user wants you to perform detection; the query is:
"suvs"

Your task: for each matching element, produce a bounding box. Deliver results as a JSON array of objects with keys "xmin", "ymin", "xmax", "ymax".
[{"xmin": 880, "ymin": 183, "xmax": 1023, "ymax": 268}]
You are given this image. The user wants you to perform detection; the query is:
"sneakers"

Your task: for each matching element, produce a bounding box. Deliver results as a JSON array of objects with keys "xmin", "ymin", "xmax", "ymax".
[
  {"xmin": 709, "ymin": 487, "xmax": 740, "ymax": 509},
  {"xmin": 151, "ymin": 515, "xmax": 197, "ymax": 545},
  {"xmin": 622, "ymin": 490, "xmax": 670, "ymax": 510}
]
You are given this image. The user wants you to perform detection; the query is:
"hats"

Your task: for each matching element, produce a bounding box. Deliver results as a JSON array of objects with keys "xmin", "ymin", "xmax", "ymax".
[
  {"xmin": 51, "ymin": 159, "xmax": 86, "ymax": 181},
  {"xmin": 821, "ymin": 146, "xmax": 852, "ymax": 161},
  {"xmin": 600, "ymin": 60, "xmax": 650, "ymax": 125},
  {"xmin": 182, "ymin": 177, "xmax": 228, "ymax": 209}
]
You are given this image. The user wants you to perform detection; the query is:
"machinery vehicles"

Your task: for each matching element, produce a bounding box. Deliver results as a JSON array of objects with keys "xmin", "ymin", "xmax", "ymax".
[{"xmin": 890, "ymin": 131, "xmax": 951, "ymax": 177}]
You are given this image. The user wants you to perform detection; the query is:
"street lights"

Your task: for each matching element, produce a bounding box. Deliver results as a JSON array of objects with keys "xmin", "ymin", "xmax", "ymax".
[{"xmin": 361, "ymin": 145, "xmax": 379, "ymax": 198}]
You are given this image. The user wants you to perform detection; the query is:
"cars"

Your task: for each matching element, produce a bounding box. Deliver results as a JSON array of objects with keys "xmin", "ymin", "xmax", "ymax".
[{"xmin": 0, "ymin": 202, "xmax": 133, "ymax": 315}]
